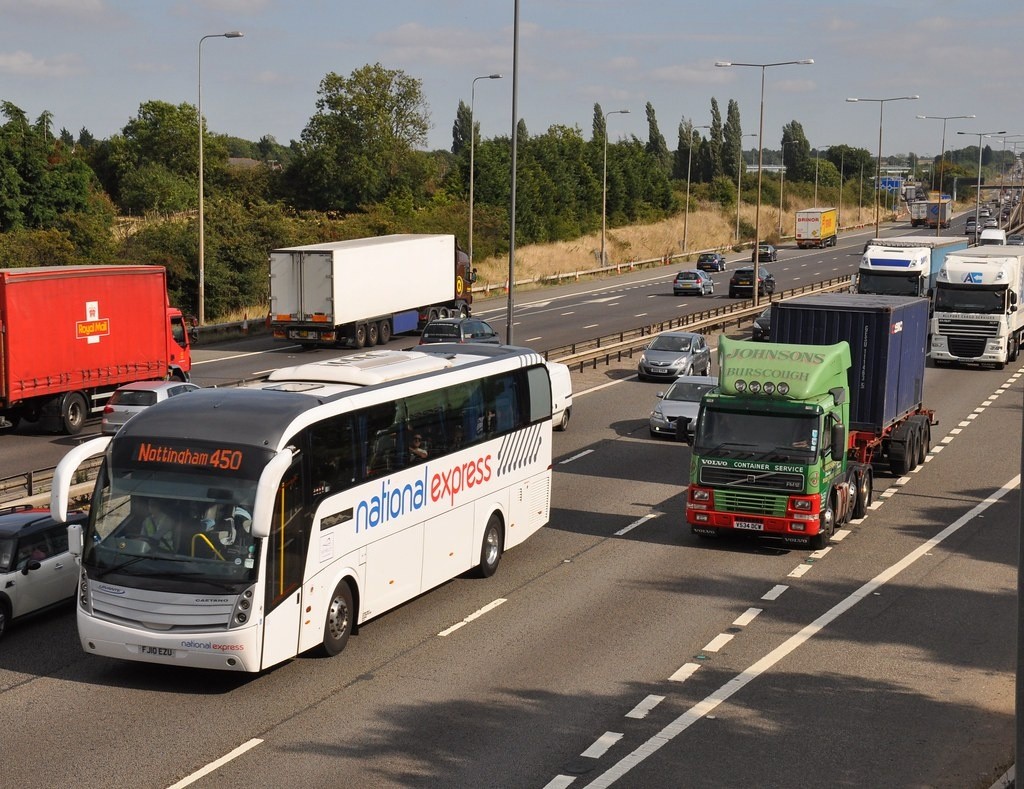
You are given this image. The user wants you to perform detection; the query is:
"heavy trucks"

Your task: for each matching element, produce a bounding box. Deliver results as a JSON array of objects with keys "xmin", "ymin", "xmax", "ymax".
[
  {"xmin": 849, "ymin": 235, "xmax": 969, "ymax": 320},
  {"xmin": 675, "ymin": 291, "xmax": 942, "ymax": 551},
  {"xmin": 924, "ymin": 244, "xmax": 1024, "ymax": 370},
  {"xmin": 266, "ymin": 233, "xmax": 477, "ymax": 349}
]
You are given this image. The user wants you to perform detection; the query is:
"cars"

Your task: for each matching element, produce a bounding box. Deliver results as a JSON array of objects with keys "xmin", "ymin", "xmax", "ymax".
[
  {"xmin": 102, "ymin": 379, "xmax": 218, "ymax": 435},
  {"xmin": 752, "ymin": 305, "xmax": 771, "ymax": 342},
  {"xmin": 0, "ymin": 505, "xmax": 100, "ymax": 638},
  {"xmin": 673, "ymin": 268, "xmax": 715, "ymax": 297},
  {"xmin": 698, "ymin": 252, "xmax": 727, "ymax": 272},
  {"xmin": 966, "ymin": 188, "xmax": 1024, "ymax": 246},
  {"xmin": 638, "ymin": 330, "xmax": 712, "ymax": 380},
  {"xmin": 648, "ymin": 376, "xmax": 719, "ymax": 437},
  {"xmin": 752, "ymin": 245, "xmax": 778, "ymax": 263}
]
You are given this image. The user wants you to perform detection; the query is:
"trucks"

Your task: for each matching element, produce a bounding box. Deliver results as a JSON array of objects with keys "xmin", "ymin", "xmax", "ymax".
[
  {"xmin": 795, "ymin": 207, "xmax": 841, "ymax": 246},
  {"xmin": 0, "ymin": 265, "xmax": 198, "ymax": 435}
]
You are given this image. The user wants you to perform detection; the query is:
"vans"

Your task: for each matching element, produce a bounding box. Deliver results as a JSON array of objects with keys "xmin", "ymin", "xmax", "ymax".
[{"xmin": 545, "ymin": 361, "xmax": 573, "ymax": 432}]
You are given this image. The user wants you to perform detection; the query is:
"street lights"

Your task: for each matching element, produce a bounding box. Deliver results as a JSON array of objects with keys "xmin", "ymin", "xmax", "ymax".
[
  {"xmin": 847, "ymin": 95, "xmax": 920, "ymax": 237},
  {"xmin": 602, "ymin": 109, "xmax": 631, "ymax": 267},
  {"xmin": 957, "ymin": 130, "xmax": 1006, "ymax": 247},
  {"xmin": 996, "ymin": 140, "xmax": 1024, "ymax": 231},
  {"xmin": 682, "ymin": 125, "xmax": 713, "ymax": 253},
  {"xmin": 984, "ymin": 133, "xmax": 1024, "ymax": 230},
  {"xmin": 714, "ymin": 59, "xmax": 815, "ymax": 304},
  {"xmin": 916, "ymin": 114, "xmax": 977, "ymax": 236},
  {"xmin": 735, "ymin": 133, "xmax": 758, "ymax": 240},
  {"xmin": 198, "ymin": 31, "xmax": 246, "ymax": 325},
  {"xmin": 469, "ymin": 73, "xmax": 504, "ymax": 276}
]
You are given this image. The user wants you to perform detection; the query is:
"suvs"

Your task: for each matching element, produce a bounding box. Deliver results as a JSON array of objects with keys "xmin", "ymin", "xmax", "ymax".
[
  {"xmin": 729, "ymin": 265, "xmax": 776, "ymax": 298},
  {"xmin": 418, "ymin": 316, "xmax": 501, "ymax": 345}
]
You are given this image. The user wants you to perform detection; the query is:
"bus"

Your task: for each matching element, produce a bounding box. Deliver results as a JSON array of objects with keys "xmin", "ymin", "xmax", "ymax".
[{"xmin": 49, "ymin": 341, "xmax": 552, "ymax": 673}]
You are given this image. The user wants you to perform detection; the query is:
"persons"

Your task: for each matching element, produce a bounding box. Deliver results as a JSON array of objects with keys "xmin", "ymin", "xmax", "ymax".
[
  {"xmin": 18, "ymin": 543, "xmax": 45, "ymax": 567},
  {"xmin": 139, "ymin": 498, "xmax": 173, "ymax": 550},
  {"xmin": 409, "ymin": 433, "xmax": 427, "ymax": 460}
]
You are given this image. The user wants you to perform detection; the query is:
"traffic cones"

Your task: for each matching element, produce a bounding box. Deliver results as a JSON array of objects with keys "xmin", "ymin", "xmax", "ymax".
[
  {"xmin": 616, "ymin": 261, "xmax": 621, "ymax": 274},
  {"xmin": 556, "ymin": 270, "xmax": 562, "ymax": 287},
  {"xmin": 240, "ymin": 313, "xmax": 249, "ymax": 338},
  {"xmin": 503, "ymin": 278, "xmax": 510, "ymax": 294},
  {"xmin": 484, "ymin": 279, "xmax": 491, "ymax": 296},
  {"xmin": 629, "ymin": 258, "xmax": 634, "ymax": 271},
  {"xmin": 574, "ymin": 268, "xmax": 580, "ymax": 282}
]
